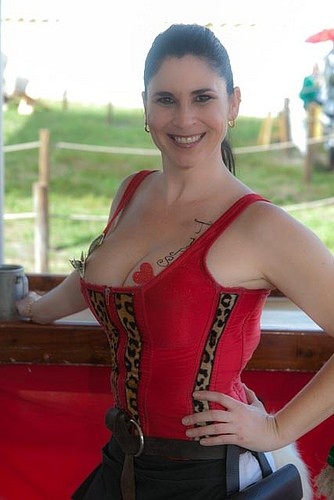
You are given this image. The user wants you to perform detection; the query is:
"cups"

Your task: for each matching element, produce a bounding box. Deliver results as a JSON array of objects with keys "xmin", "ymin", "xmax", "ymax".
[{"xmin": 0, "ymin": 264, "xmax": 29, "ymax": 321}]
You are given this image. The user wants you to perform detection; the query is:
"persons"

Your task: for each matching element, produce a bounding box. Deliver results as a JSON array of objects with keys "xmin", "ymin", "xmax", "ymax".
[{"xmin": 13, "ymin": 22, "xmax": 334, "ymax": 500}]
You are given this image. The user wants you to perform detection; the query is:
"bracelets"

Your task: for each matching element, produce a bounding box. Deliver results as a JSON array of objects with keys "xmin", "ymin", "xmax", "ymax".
[{"xmin": 27, "ymin": 295, "xmax": 43, "ymax": 320}]
